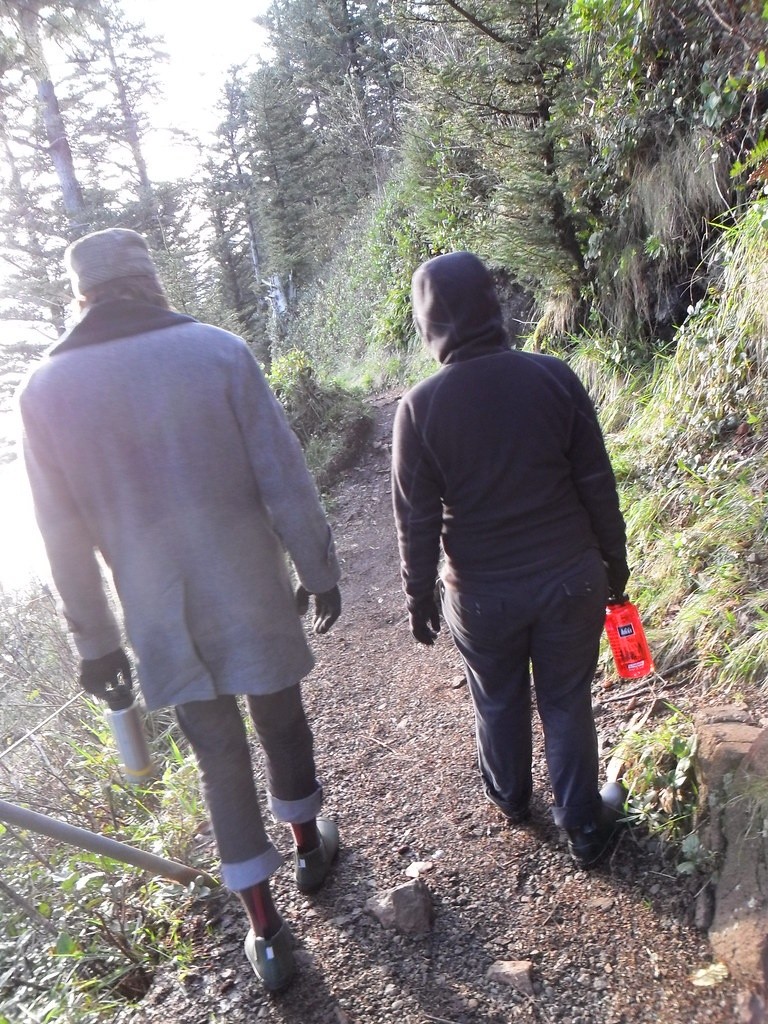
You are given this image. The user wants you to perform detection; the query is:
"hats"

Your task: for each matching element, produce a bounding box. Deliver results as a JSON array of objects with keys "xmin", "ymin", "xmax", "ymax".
[{"xmin": 64, "ymin": 227, "xmax": 155, "ymax": 294}]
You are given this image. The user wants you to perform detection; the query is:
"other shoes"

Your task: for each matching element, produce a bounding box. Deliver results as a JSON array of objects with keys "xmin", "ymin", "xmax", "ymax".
[
  {"xmin": 244, "ymin": 928, "xmax": 292, "ymax": 993},
  {"xmin": 295, "ymin": 816, "xmax": 339, "ymax": 891}
]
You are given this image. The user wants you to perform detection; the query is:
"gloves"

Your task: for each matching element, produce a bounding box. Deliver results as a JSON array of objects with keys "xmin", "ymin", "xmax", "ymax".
[
  {"xmin": 608, "ymin": 560, "xmax": 631, "ymax": 603},
  {"xmin": 406, "ymin": 594, "xmax": 441, "ymax": 647}
]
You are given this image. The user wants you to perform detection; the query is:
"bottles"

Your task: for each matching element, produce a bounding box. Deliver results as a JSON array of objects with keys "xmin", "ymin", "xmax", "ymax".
[
  {"xmin": 103, "ymin": 684, "xmax": 153, "ymax": 783},
  {"xmin": 606, "ymin": 587, "xmax": 651, "ymax": 679}
]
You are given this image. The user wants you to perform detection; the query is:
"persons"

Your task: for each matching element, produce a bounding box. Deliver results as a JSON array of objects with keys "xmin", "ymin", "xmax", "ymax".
[
  {"xmin": 13, "ymin": 229, "xmax": 344, "ymax": 995},
  {"xmin": 391, "ymin": 252, "xmax": 635, "ymax": 870}
]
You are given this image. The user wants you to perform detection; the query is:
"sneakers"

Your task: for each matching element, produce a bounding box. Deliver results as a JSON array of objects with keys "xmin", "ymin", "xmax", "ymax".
[
  {"xmin": 568, "ymin": 781, "xmax": 628, "ymax": 871},
  {"xmin": 500, "ymin": 804, "xmax": 532, "ymax": 823}
]
